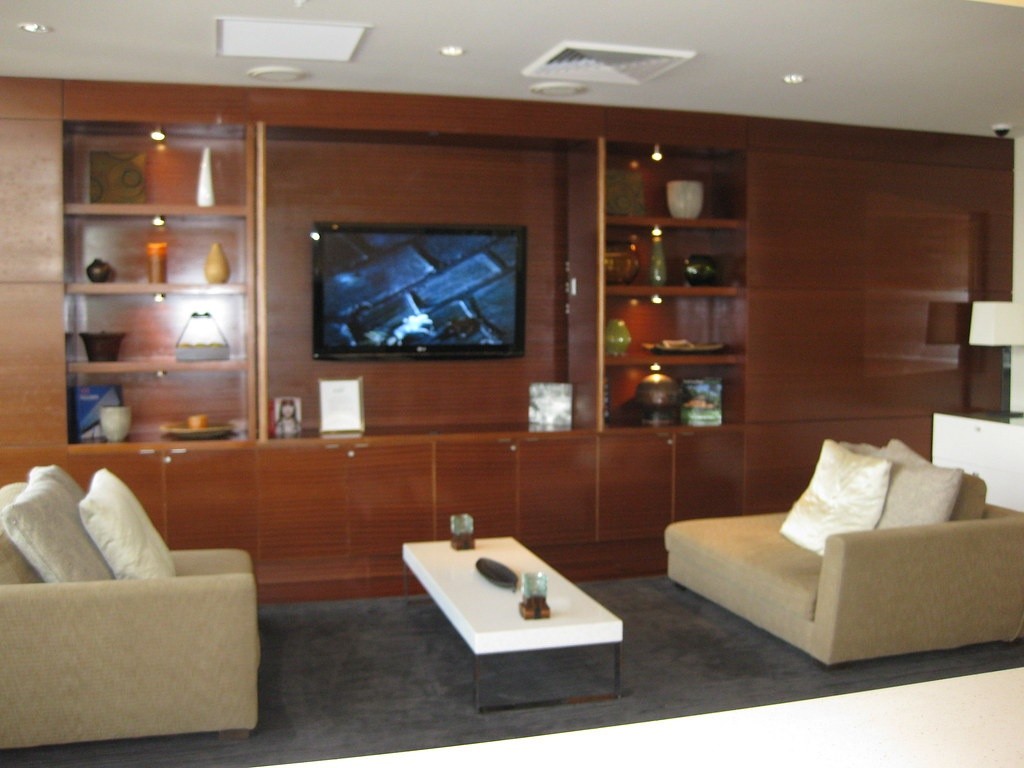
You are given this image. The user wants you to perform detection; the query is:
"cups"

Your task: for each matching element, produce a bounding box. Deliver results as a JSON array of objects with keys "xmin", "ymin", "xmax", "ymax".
[
  {"xmin": 148, "ymin": 242, "xmax": 167, "ymax": 284},
  {"xmin": 666, "ymin": 180, "xmax": 703, "ymax": 219},
  {"xmin": 450, "ymin": 514, "xmax": 476, "ymax": 550},
  {"xmin": 100, "ymin": 405, "xmax": 131, "ymax": 443},
  {"xmin": 520, "ymin": 571, "xmax": 548, "ymax": 611}
]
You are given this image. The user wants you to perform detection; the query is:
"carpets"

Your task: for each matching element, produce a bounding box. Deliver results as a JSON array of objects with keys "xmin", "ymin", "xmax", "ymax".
[{"xmin": 0, "ymin": 574, "xmax": 1024, "ymax": 768}]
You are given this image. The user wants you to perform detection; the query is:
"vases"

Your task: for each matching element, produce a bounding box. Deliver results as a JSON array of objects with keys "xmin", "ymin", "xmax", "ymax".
[
  {"xmin": 102, "ymin": 406, "xmax": 131, "ymax": 445},
  {"xmin": 78, "ymin": 332, "xmax": 125, "ymax": 363},
  {"xmin": 204, "ymin": 242, "xmax": 228, "ymax": 284}
]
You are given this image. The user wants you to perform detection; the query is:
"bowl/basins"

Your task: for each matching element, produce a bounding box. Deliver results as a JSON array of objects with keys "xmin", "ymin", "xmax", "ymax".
[{"xmin": 79, "ymin": 332, "xmax": 126, "ymax": 362}]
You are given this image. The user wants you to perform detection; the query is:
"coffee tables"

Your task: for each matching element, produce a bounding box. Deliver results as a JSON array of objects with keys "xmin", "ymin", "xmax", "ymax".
[{"xmin": 403, "ymin": 537, "xmax": 625, "ymax": 716}]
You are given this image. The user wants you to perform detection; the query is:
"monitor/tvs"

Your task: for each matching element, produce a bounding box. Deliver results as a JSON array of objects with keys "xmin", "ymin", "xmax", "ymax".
[{"xmin": 310, "ymin": 222, "xmax": 528, "ymax": 360}]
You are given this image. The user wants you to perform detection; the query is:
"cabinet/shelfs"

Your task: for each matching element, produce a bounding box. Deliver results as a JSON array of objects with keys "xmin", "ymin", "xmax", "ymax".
[{"xmin": 0, "ymin": 75, "xmax": 1017, "ymax": 598}]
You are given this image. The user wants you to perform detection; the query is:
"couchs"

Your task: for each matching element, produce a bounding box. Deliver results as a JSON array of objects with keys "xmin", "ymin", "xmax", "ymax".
[
  {"xmin": 665, "ymin": 503, "xmax": 1024, "ymax": 665},
  {"xmin": 1, "ymin": 480, "xmax": 263, "ymax": 749}
]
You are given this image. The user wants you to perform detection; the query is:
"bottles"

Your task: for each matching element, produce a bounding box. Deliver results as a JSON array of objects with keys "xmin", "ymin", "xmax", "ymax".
[
  {"xmin": 86, "ymin": 258, "xmax": 111, "ymax": 282},
  {"xmin": 685, "ymin": 253, "xmax": 720, "ymax": 287},
  {"xmin": 650, "ymin": 237, "xmax": 667, "ymax": 287},
  {"xmin": 605, "ymin": 318, "xmax": 631, "ymax": 355},
  {"xmin": 204, "ymin": 243, "xmax": 230, "ymax": 284}
]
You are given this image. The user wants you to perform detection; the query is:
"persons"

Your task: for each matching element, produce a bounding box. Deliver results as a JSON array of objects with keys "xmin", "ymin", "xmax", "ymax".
[{"xmin": 274, "ymin": 399, "xmax": 301, "ymax": 438}]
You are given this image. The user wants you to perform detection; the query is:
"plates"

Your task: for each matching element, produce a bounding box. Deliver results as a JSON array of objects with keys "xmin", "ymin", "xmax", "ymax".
[
  {"xmin": 641, "ymin": 341, "xmax": 723, "ymax": 353},
  {"xmin": 160, "ymin": 422, "xmax": 236, "ymax": 439}
]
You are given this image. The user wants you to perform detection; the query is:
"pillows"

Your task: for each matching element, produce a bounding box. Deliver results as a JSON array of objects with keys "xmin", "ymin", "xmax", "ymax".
[
  {"xmin": 779, "ymin": 439, "xmax": 987, "ymax": 555},
  {"xmin": 0, "ymin": 461, "xmax": 179, "ymax": 583}
]
